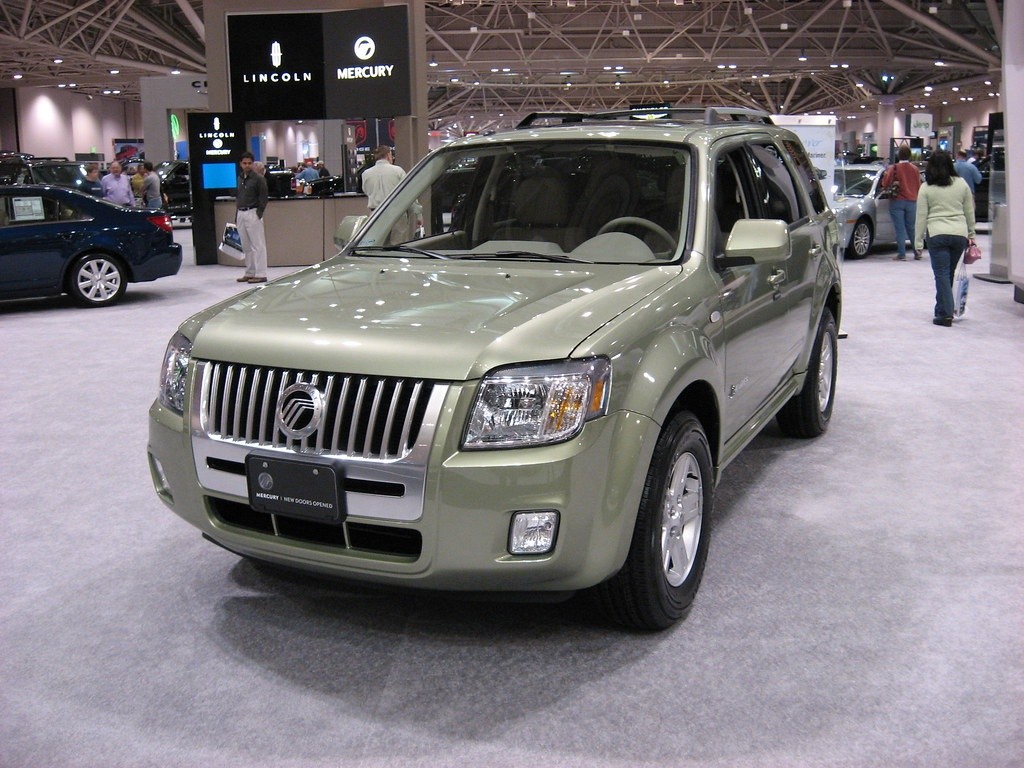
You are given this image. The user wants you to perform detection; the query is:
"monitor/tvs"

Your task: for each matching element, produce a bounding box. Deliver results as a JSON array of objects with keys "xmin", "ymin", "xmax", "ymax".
[
  {"xmin": 202, "ymin": 163, "xmax": 237, "ymax": 189},
  {"xmin": 265, "ymin": 173, "xmax": 297, "ymax": 198}
]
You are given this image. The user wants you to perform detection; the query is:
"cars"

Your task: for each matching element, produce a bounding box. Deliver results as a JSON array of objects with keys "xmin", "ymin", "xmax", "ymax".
[
  {"xmin": 150, "ymin": 161, "xmax": 191, "ymax": 218},
  {"xmin": 816, "ymin": 164, "xmax": 897, "ymax": 260},
  {"xmin": 965, "ymin": 144, "xmax": 1004, "ymax": 223},
  {"xmin": 0, "ymin": 183, "xmax": 184, "ymax": 308}
]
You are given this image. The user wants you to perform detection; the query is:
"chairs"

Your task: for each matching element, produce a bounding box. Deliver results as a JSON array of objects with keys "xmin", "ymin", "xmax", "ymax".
[
  {"xmin": 643, "ymin": 162, "xmax": 729, "ymax": 251},
  {"xmin": 493, "ymin": 175, "xmax": 587, "ymax": 253}
]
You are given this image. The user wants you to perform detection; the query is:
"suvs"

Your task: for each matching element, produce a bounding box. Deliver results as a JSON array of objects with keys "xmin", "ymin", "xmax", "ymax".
[
  {"xmin": 146, "ymin": 103, "xmax": 849, "ymax": 636},
  {"xmin": 0, "ymin": 151, "xmax": 145, "ymax": 212}
]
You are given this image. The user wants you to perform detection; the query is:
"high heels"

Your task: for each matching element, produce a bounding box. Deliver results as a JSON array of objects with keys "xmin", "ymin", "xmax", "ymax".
[{"xmin": 933, "ymin": 317, "xmax": 952, "ymax": 327}]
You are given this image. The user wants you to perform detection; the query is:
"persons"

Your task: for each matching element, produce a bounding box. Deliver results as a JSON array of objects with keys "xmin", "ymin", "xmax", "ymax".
[
  {"xmin": 100, "ymin": 160, "xmax": 136, "ymax": 209},
  {"xmin": 914, "ymin": 149, "xmax": 975, "ymax": 327},
  {"xmin": 881, "ymin": 145, "xmax": 923, "ymax": 261},
  {"xmin": 836, "ymin": 153, "xmax": 847, "ymax": 165},
  {"xmin": 316, "ymin": 161, "xmax": 330, "ymax": 177},
  {"xmin": 295, "ymin": 162, "xmax": 319, "ymax": 182},
  {"xmin": 297, "ymin": 162, "xmax": 306, "ymax": 173},
  {"xmin": 235, "ymin": 152, "xmax": 269, "ymax": 284},
  {"xmin": 253, "ymin": 161, "xmax": 279, "ymax": 197},
  {"xmin": 122, "ymin": 162, "xmax": 169, "ymax": 209},
  {"xmin": 362, "ymin": 145, "xmax": 423, "ymax": 245},
  {"xmin": 953, "ymin": 150, "xmax": 982, "ymax": 196},
  {"xmin": 81, "ymin": 163, "xmax": 104, "ymax": 197}
]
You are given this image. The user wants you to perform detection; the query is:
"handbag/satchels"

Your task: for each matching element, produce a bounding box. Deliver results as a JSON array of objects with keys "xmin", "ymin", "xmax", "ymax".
[
  {"xmin": 414, "ymin": 226, "xmax": 426, "ymax": 240},
  {"xmin": 951, "ymin": 262, "xmax": 969, "ymax": 317},
  {"xmin": 878, "ymin": 163, "xmax": 899, "ymax": 200},
  {"xmin": 219, "ymin": 222, "xmax": 245, "ymax": 260},
  {"xmin": 963, "ymin": 243, "xmax": 981, "ymax": 264}
]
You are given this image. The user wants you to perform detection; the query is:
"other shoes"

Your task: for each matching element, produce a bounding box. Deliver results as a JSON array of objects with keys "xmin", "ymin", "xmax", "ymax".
[
  {"xmin": 248, "ymin": 277, "xmax": 267, "ymax": 283},
  {"xmin": 236, "ymin": 276, "xmax": 252, "ymax": 282},
  {"xmin": 892, "ymin": 255, "xmax": 907, "ymax": 261}
]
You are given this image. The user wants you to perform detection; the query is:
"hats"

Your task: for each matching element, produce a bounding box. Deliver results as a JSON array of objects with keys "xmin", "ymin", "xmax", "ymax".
[{"xmin": 316, "ymin": 160, "xmax": 324, "ymax": 164}]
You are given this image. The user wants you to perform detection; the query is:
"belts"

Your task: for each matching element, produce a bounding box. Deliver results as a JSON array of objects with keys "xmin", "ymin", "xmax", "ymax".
[{"xmin": 237, "ymin": 206, "xmax": 257, "ymax": 211}]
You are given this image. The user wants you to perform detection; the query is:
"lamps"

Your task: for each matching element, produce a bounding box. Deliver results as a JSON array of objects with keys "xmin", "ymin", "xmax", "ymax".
[
  {"xmin": 934, "ymin": 53, "xmax": 945, "ymax": 66},
  {"xmin": 106, "ymin": 68, "xmax": 120, "ymax": 74},
  {"xmin": 797, "ymin": 47, "xmax": 808, "ymax": 62}
]
